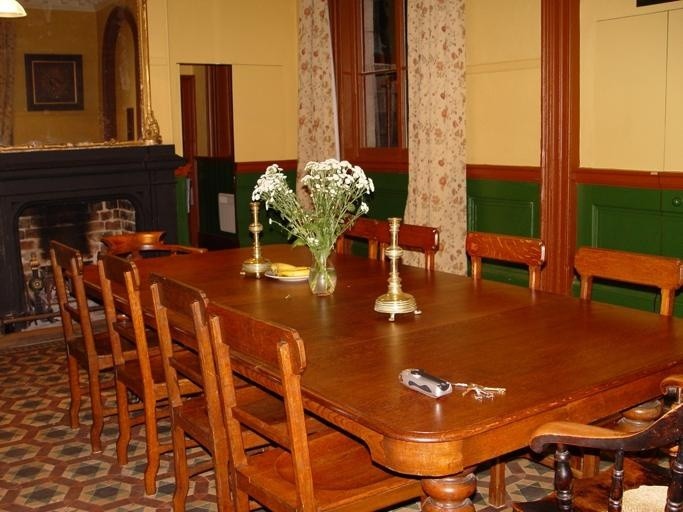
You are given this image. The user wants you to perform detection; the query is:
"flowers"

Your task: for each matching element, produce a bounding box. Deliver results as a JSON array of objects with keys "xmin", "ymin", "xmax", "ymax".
[{"xmin": 251, "ymin": 159, "xmax": 375, "ymax": 288}]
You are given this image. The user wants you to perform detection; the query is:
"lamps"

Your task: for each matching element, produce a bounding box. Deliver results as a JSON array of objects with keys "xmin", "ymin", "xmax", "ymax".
[{"xmin": 0, "ymin": 0, "xmax": 27, "ymax": 18}]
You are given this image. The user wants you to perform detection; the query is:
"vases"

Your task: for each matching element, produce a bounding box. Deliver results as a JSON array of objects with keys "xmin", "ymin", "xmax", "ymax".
[{"xmin": 308, "ymin": 248, "xmax": 337, "ymax": 297}]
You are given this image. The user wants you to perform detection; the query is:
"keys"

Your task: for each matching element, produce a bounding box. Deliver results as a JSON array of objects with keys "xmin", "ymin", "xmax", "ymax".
[{"xmin": 461, "ymin": 383, "xmax": 507, "ymax": 401}]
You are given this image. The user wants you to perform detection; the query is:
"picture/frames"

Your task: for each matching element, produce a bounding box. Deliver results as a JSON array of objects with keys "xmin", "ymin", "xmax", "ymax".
[{"xmin": 23, "ymin": 53, "xmax": 85, "ymax": 112}]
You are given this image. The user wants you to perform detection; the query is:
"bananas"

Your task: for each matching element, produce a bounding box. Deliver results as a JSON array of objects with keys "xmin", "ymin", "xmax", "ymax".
[{"xmin": 271, "ymin": 263, "xmax": 311, "ymax": 276}]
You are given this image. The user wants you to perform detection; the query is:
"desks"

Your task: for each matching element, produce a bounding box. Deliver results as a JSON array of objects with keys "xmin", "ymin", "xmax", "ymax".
[{"xmin": 66, "ymin": 244, "xmax": 683, "ymax": 510}]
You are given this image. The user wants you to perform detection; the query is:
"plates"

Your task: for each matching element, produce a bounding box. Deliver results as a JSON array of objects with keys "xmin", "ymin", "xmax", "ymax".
[{"xmin": 264, "ymin": 270, "xmax": 309, "ymax": 283}]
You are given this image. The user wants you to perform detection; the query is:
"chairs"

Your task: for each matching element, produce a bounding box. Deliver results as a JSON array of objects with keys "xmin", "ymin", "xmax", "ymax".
[
  {"xmin": 99, "ymin": 229, "xmax": 209, "ymax": 320},
  {"xmin": 335, "ymin": 211, "xmax": 440, "ymax": 272},
  {"xmin": 511, "ymin": 370, "xmax": 683, "ymax": 511},
  {"xmin": 47, "ymin": 239, "xmax": 182, "ymax": 455},
  {"xmin": 149, "ymin": 268, "xmax": 328, "ymax": 512},
  {"xmin": 97, "ymin": 250, "xmax": 249, "ymax": 496},
  {"xmin": 488, "ymin": 246, "xmax": 683, "ymax": 509},
  {"xmin": 207, "ymin": 299, "xmax": 423, "ymax": 511},
  {"xmin": 465, "ymin": 230, "xmax": 547, "ymax": 292}
]
